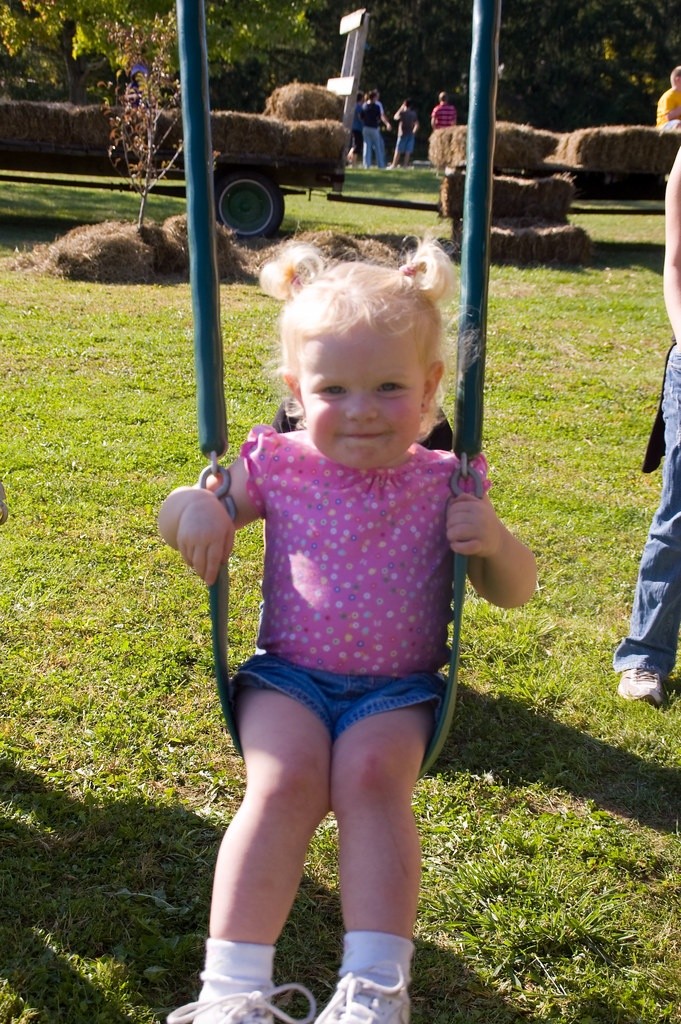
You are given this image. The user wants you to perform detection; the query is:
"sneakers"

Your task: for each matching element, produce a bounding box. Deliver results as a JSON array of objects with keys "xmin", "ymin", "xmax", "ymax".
[
  {"xmin": 167, "ymin": 984, "xmax": 313, "ymax": 1024},
  {"xmin": 617, "ymin": 667, "xmax": 664, "ymax": 710},
  {"xmin": 314, "ymin": 967, "xmax": 411, "ymax": 1024}
]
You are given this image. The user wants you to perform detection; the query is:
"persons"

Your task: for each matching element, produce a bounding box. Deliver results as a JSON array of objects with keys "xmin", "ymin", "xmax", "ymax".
[
  {"xmin": 657, "ymin": 66, "xmax": 681, "ymax": 131},
  {"xmin": 386, "ymin": 100, "xmax": 420, "ymax": 169},
  {"xmin": 612, "ymin": 148, "xmax": 680, "ymax": 705},
  {"xmin": 346, "ymin": 90, "xmax": 392, "ymax": 169},
  {"xmin": 431, "ymin": 92, "xmax": 456, "ymax": 131},
  {"xmin": 158, "ymin": 242, "xmax": 537, "ymax": 1024}
]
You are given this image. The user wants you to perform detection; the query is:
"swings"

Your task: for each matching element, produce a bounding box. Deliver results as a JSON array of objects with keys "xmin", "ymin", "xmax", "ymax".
[{"xmin": 175, "ymin": 0, "xmax": 504, "ymax": 782}]
